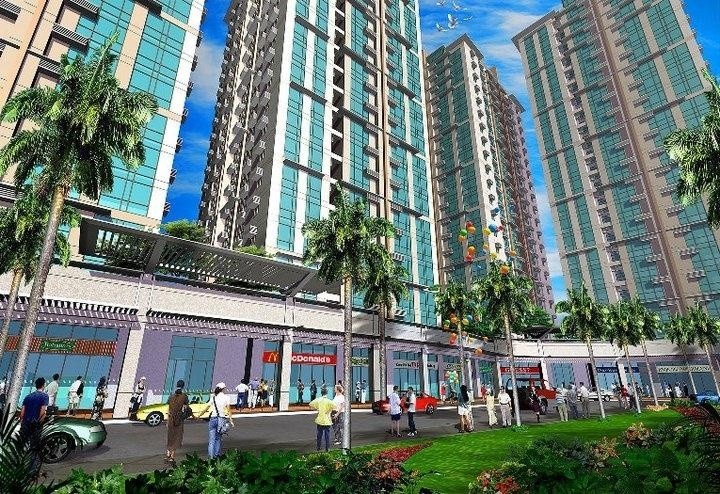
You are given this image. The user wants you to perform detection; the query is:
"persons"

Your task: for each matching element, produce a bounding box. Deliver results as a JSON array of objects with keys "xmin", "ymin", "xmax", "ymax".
[
  {"xmin": 235, "ymin": 377, "xmax": 276, "ymax": 408},
  {"xmin": 19, "ymin": 377, "xmax": 49, "ymax": 469},
  {"xmin": 296, "ymin": 378, "xmax": 345, "ymax": 452},
  {"xmin": 90, "ymin": 376, "xmax": 108, "ymax": 420},
  {"xmin": 355, "ymin": 380, "xmax": 367, "ymax": 404},
  {"xmin": 389, "ymin": 385, "xmax": 417, "ymax": 436},
  {"xmin": 0, "ymin": 377, "xmax": 7, "ymax": 404},
  {"xmin": 661, "ymin": 381, "xmax": 688, "ymax": 402},
  {"xmin": 44, "ymin": 373, "xmax": 59, "ymax": 406},
  {"xmin": 611, "ymin": 382, "xmax": 650, "ymax": 410},
  {"xmin": 66, "ymin": 376, "xmax": 83, "ymax": 415},
  {"xmin": 130, "ymin": 376, "xmax": 146, "ymax": 421},
  {"xmin": 164, "ymin": 380, "xmax": 200, "ymax": 462},
  {"xmin": 528, "ymin": 381, "xmax": 590, "ymax": 422},
  {"xmin": 196, "ymin": 382, "xmax": 234, "ymax": 458},
  {"xmin": 441, "ymin": 380, "xmax": 512, "ymax": 433}
]
